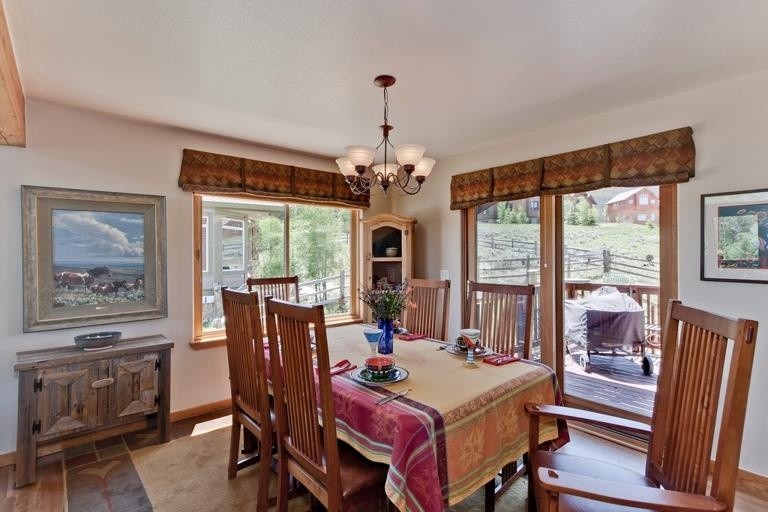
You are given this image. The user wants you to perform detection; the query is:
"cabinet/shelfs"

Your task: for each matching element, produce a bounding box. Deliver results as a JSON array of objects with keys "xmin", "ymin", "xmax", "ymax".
[
  {"xmin": 359, "ymin": 214, "xmax": 417, "ymax": 325},
  {"xmin": 13, "ymin": 335, "xmax": 176, "ymax": 487}
]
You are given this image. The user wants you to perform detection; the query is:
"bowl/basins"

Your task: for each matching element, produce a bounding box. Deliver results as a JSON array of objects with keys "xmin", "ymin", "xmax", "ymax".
[
  {"xmin": 73, "ymin": 330, "xmax": 122, "ymax": 351},
  {"xmin": 363, "ymin": 357, "xmax": 394, "ymax": 378},
  {"xmin": 455, "ymin": 337, "xmax": 478, "ymax": 352},
  {"xmin": 386, "ymin": 247, "xmax": 397, "ymax": 257}
]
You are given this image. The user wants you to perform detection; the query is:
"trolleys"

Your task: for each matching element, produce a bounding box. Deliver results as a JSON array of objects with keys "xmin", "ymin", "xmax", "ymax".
[{"xmin": 565, "ymin": 285, "xmax": 654, "ymax": 376}]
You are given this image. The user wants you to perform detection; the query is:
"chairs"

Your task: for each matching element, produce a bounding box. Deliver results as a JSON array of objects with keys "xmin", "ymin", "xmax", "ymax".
[
  {"xmin": 245, "ymin": 275, "xmax": 302, "ymax": 336},
  {"xmin": 523, "ymin": 296, "xmax": 760, "ymax": 511},
  {"xmin": 219, "ymin": 283, "xmax": 319, "ymax": 511},
  {"xmin": 262, "ymin": 293, "xmax": 397, "ymax": 511},
  {"xmin": 463, "ymin": 279, "xmax": 536, "ymax": 357},
  {"xmin": 400, "ymin": 277, "xmax": 451, "ymax": 343}
]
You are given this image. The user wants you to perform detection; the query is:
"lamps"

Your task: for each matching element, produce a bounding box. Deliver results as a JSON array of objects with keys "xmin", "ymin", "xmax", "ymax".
[
  {"xmin": 357, "ymin": 274, "xmax": 418, "ymax": 320},
  {"xmin": 334, "ymin": 75, "xmax": 436, "ymax": 198}
]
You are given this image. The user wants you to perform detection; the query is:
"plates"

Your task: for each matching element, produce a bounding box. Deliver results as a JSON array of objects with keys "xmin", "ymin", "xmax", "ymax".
[
  {"xmin": 444, "ymin": 343, "xmax": 493, "ymax": 356},
  {"xmin": 349, "ymin": 366, "xmax": 409, "ymax": 386},
  {"xmin": 358, "ymin": 367, "xmax": 400, "ymax": 382}
]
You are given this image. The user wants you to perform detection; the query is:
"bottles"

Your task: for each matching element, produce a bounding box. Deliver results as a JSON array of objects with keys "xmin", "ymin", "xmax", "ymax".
[{"xmin": 372, "ymin": 276, "xmax": 401, "ymax": 292}]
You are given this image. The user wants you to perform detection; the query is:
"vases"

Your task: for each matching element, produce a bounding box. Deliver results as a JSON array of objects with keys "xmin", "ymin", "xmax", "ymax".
[{"xmin": 376, "ymin": 316, "xmax": 394, "ymax": 354}]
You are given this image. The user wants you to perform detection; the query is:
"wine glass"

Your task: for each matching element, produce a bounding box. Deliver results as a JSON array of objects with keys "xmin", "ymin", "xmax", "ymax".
[
  {"xmin": 362, "ymin": 329, "xmax": 383, "ymax": 358},
  {"xmin": 456, "ymin": 329, "xmax": 480, "ymax": 370}
]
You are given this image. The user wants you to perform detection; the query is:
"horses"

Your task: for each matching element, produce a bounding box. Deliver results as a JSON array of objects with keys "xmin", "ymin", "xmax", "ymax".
[{"xmin": 54, "ymin": 266, "xmax": 144, "ymax": 298}]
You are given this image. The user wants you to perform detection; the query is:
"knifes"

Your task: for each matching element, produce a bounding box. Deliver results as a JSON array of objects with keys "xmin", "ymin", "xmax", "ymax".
[{"xmin": 373, "ymin": 386, "xmax": 412, "ymax": 407}]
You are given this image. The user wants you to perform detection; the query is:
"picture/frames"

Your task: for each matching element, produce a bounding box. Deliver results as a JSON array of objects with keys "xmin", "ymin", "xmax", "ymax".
[
  {"xmin": 699, "ymin": 189, "xmax": 767, "ymax": 285},
  {"xmin": 20, "ymin": 184, "xmax": 169, "ymax": 334}
]
